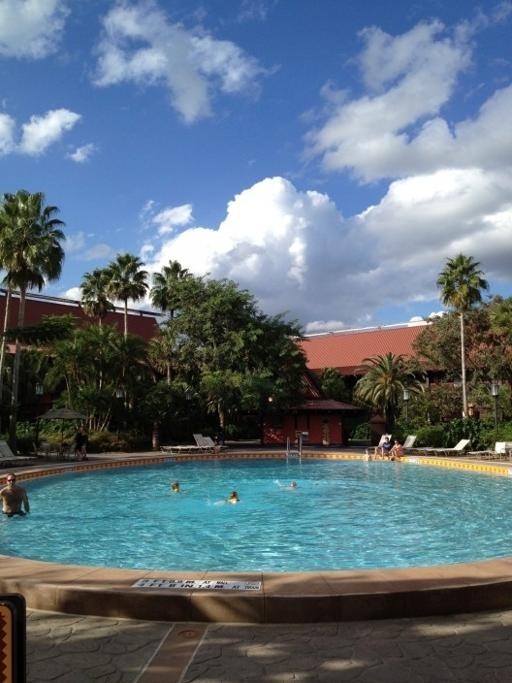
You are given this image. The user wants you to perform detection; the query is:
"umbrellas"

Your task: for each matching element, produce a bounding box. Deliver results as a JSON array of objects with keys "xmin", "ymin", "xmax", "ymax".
[{"xmin": 35, "ymin": 406, "xmax": 90, "ymax": 448}]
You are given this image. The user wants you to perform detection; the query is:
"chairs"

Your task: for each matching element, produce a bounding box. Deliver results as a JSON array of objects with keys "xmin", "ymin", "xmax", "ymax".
[
  {"xmin": 161, "ymin": 433, "xmax": 228, "ymax": 454},
  {"xmin": 364, "ymin": 433, "xmax": 512, "ymax": 466},
  {"xmin": 0, "ymin": 440, "xmax": 37, "ymax": 468}
]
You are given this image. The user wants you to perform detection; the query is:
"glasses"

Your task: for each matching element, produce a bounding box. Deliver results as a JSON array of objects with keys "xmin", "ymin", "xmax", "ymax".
[{"xmin": 6, "ymin": 479, "xmax": 15, "ymax": 482}]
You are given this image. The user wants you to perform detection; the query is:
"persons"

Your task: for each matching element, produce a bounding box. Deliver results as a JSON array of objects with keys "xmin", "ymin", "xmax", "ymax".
[
  {"xmin": 0, "ymin": 472, "xmax": 30, "ymax": 517},
  {"xmin": 387, "ymin": 438, "xmax": 405, "ymax": 460},
  {"xmin": 373, "ymin": 435, "xmax": 393, "ymax": 460},
  {"xmin": 228, "ymin": 491, "xmax": 240, "ymax": 503},
  {"xmin": 362, "ymin": 447, "xmax": 373, "ymax": 461},
  {"xmin": 170, "ymin": 481, "xmax": 180, "ymax": 492},
  {"xmin": 289, "ymin": 481, "xmax": 297, "ymax": 489},
  {"xmin": 74, "ymin": 424, "xmax": 89, "ymax": 461}
]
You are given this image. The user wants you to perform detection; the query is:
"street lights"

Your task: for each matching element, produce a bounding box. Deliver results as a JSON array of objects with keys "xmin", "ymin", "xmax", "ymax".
[
  {"xmin": 403, "ymin": 388, "xmax": 411, "ymax": 422},
  {"xmin": 35, "ymin": 381, "xmax": 45, "ymax": 448},
  {"xmin": 185, "ymin": 389, "xmax": 192, "ymax": 419},
  {"xmin": 491, "ymin": 382, "xmax": 500, "ymax": 433},
  {"xmin": 115, "ymin": 386, "xmax": 123, "ymax": 442}
]
